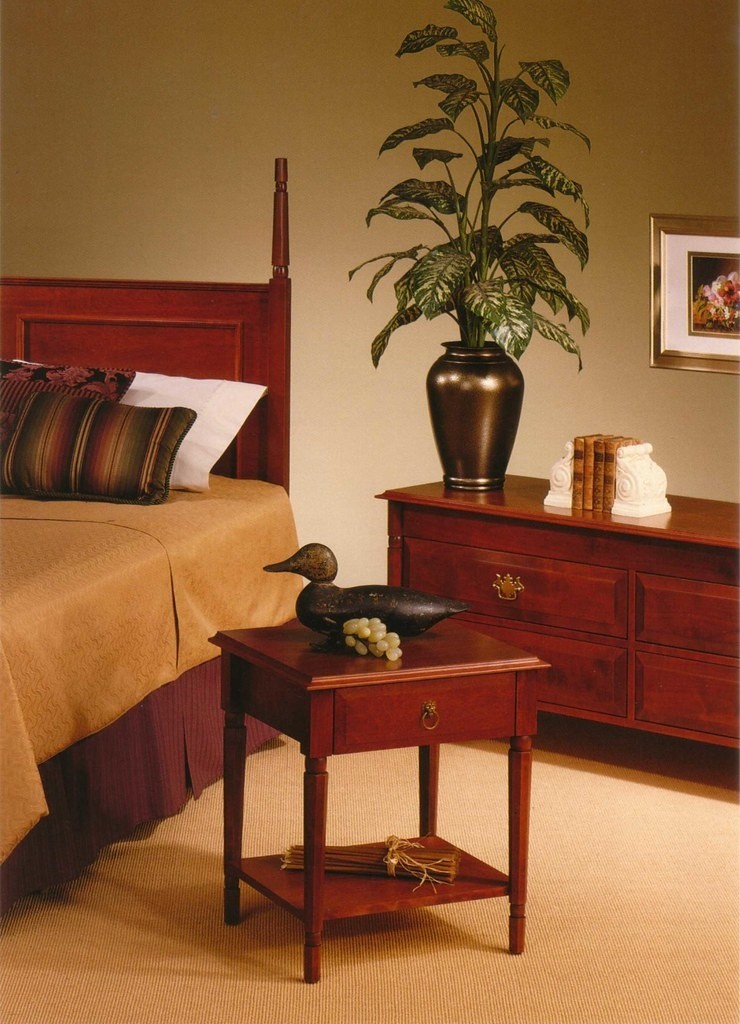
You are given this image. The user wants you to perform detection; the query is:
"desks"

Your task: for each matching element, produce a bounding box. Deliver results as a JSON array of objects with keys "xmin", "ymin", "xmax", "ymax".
[{"xmin": 206, "ymin": 617, "xmax": 551, "ymax": 984}]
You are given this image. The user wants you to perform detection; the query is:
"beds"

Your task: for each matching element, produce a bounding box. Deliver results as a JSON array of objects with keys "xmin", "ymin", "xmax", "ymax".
[{"xmin": 0, "ymin": 158, "xmax": 309, "ymax": 919}]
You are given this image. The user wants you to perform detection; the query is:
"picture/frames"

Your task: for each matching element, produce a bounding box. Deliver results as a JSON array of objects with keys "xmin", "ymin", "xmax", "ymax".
[{"xmin": 647, "ymin": 212, "xmax": 740, "ymax": 376}]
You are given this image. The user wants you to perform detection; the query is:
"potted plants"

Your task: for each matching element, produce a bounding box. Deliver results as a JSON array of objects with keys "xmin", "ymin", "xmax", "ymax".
[{"xmin": 346, "ymin": 1, "xmax": 591, "ymax": 490}]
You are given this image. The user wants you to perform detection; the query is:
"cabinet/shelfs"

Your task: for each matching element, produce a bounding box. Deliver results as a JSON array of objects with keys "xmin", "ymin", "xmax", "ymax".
[{"xmin": 370, "ymin": 474, "xmax": 740, "ymax": 751}]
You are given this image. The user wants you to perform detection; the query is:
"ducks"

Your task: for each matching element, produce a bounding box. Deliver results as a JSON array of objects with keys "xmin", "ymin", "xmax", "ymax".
[{"xmin": 260, "ymin": 543, "xmax": 474, "ymax": 648}]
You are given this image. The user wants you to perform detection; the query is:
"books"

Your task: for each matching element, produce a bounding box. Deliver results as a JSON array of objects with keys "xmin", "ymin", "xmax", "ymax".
[{"xmin": 571, "ymin": 434, "xmax": 642, "ymax": 514}]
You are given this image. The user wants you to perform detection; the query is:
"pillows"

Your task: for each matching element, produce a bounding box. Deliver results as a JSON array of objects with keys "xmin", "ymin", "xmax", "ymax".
[
  {"xmin": 0, "ymin": 359, "xmax": 135, "ymax": 404},
  {"xmin": 117, "ymin": 371, "xmax": 269, "ymax": 492},
  {"xmin": 0, "ymin": 390, "xmax": 196, "ymax": 505}
]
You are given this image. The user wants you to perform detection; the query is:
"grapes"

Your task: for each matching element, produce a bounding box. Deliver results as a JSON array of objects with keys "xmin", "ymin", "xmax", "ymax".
[{"xmin": 340, "ymin": 617, "xmax": 403, "ymax": 661}]
